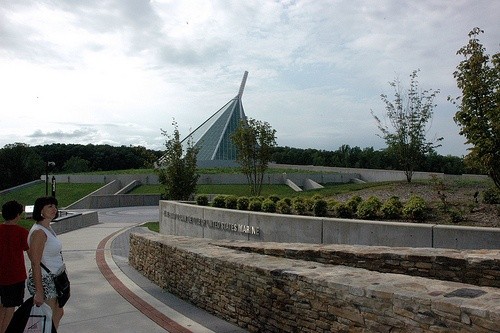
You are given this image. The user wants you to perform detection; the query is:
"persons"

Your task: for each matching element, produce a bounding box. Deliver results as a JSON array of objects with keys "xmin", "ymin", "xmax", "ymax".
[
  {"xmin": 0, "ymin": 200, "xmax": 30, "ymax": 333},
  {"xmin": 28, "ymin": 196, "xmax": 71, "ymax": 333}
]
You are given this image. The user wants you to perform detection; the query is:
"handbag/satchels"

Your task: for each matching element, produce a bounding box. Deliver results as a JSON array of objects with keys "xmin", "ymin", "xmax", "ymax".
[
  {"xmin": 40, "ymin": 262, "xmax": 70, "ymax": 308},
  {"xmin": 22, "ymin": 302, "xmax": 52, "ymax": 333},
  {"xmin": 4, "ymin": 297, "xmax": 57, "ymax": 333}
]
